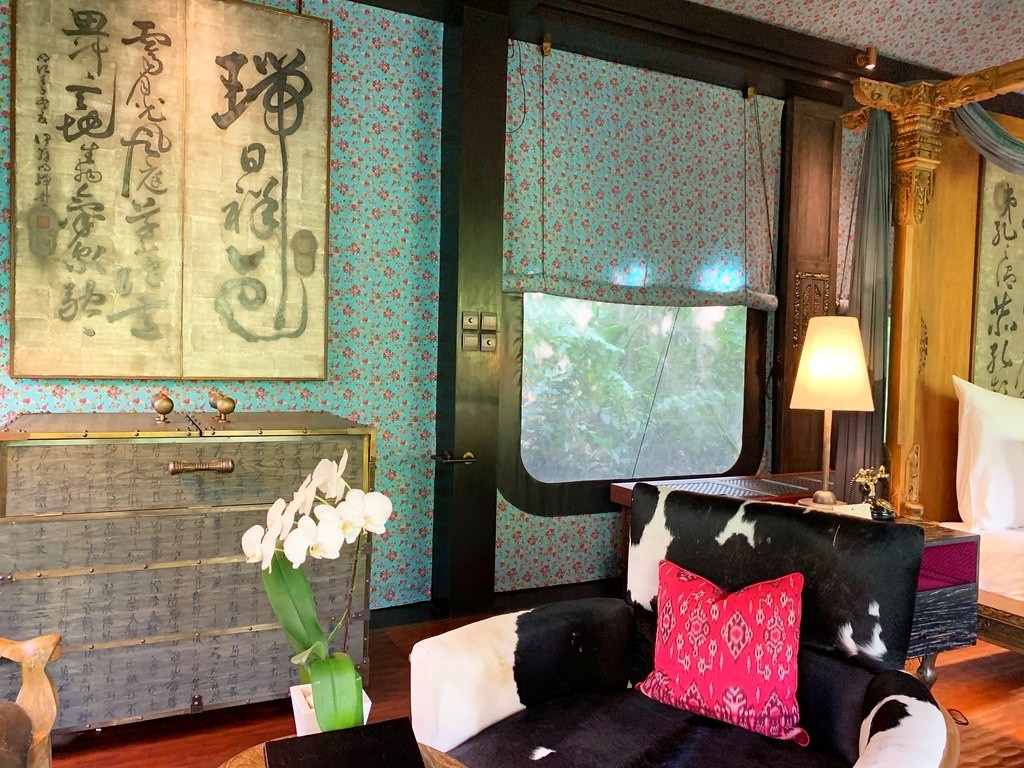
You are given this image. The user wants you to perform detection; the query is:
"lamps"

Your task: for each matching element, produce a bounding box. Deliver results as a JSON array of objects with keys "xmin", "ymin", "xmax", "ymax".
[{"xmin": 787, "ymin": 313, "xmax": 877, "ymax": 511}]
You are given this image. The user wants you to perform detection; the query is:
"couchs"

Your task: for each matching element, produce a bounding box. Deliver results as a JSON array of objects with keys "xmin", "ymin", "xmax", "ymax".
[{"xmin": 408, "ymin": 481, "xmax": 948, "ymax": 768}]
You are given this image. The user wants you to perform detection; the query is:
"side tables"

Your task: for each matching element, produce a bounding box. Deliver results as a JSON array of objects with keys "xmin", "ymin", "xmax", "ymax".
[{"xmin": 880, "ymin": 517, "xmax": 983, "ymax": 690}]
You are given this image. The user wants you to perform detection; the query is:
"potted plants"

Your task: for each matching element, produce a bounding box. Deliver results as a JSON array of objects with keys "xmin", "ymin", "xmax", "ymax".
[{"xmin": 241, "ymin": 448, "xmax": 373, "ymax": 736}]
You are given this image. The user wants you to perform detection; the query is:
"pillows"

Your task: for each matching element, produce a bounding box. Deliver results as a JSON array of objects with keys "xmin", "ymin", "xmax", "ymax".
[
  {"xmin": 952, "ymin": 374, "xmax": 1024, "ymax": 532},
  {"xmin": 631, "ymin": 560, "xmax": 811, "ymax": 748}
]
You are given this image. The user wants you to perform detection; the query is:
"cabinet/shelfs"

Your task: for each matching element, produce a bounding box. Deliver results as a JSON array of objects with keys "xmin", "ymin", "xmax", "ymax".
[{"xmin": 0, "ymin": 408, "xmax": 377, "ymax": 735}]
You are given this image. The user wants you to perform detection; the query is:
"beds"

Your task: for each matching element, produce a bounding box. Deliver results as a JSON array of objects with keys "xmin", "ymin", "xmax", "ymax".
[{"xmin": 940, "ymin": 519, "xmax": 1024, "ymax": 655}]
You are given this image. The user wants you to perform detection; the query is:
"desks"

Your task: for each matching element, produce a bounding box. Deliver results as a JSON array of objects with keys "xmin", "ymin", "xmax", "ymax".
[{"xmin": 611, "ymin": 470, "xmax": 834, "ymax": 598}]
